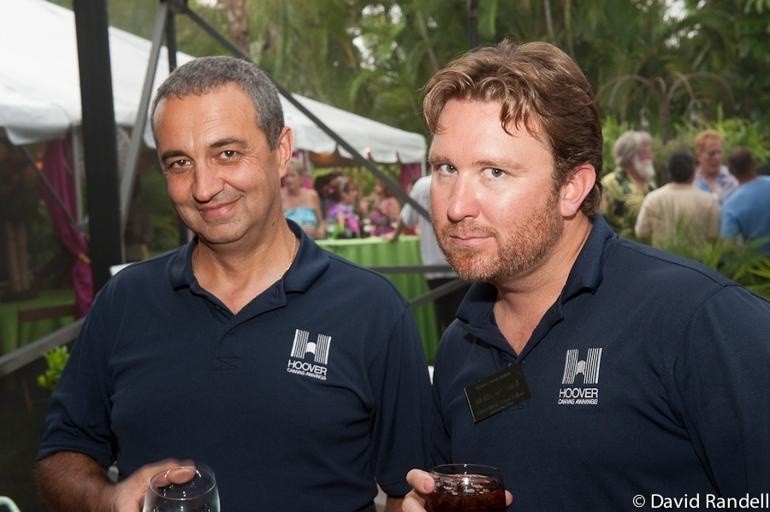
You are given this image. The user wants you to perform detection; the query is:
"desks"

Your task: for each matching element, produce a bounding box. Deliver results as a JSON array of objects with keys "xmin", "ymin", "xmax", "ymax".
[
  {"xmin": 313, "ymin": 230, "xmax": 441, "ymax": 365},
  {"xmin": 1, "ymin": 286, "xmax": 75, "ymax": 360}
]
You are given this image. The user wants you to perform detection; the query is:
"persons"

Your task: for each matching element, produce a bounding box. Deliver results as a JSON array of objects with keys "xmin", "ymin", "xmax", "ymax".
[
  {"xmin": 280, "ymin": 159, "xmax": 325, "ymax": 241},
  {"xmin": 632, "ymin": 139, "xmax": 724, "ymax": 249},
  {"xmin": 598, "ymin": 128, "xmax": 657, "ymax": 247},
  {"xmin": 398, "ymin": 168, "xmax": 469, "ymax": 326},
  {"xmin": 370, "ymin": 174, "xmax": 402, "ymax": 238},
  {"xmin": 397, "ymin": 42, "xmax": 770, "ymax": 510},
  {"xmin": 720, "ymin": 146, "xmax": 770, "ymax": 257},
  {"xmin": 322, "ymin": 173, "xmax": 360, "ymax": 237},
  {"xmin": 35, "ymin": 59, "xmax": 445, "ymax": 508},
  {"xmin": 689, "ymin": 129, "xmax": 741, "ymax": 205}
]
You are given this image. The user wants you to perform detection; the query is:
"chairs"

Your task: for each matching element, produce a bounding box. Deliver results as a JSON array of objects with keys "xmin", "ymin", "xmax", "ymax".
[{"xmin": 10, "ymin": 303, "xmax": 84, "ymax": 420}]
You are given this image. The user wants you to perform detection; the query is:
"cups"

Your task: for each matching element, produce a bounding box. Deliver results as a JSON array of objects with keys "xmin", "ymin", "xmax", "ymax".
[
  {"xmin": 142, "ymin": 463, "xmax": 220, "ymax": 512},
  {"xmin": 424, "ymin": 463, "xmax": 508, "ymax": 512}
]
